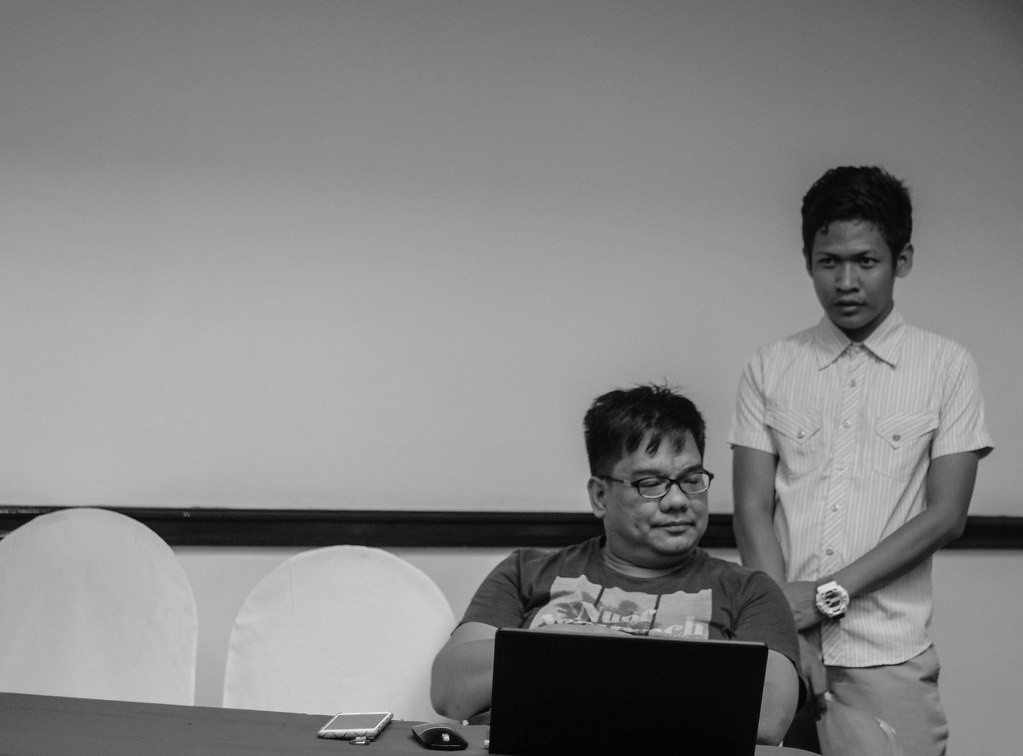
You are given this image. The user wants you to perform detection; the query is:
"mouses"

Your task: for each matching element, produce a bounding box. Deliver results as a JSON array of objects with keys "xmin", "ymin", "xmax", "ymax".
[{"xmin": 412, "ymin": 722, "xmax": 469, "ymax": 750}]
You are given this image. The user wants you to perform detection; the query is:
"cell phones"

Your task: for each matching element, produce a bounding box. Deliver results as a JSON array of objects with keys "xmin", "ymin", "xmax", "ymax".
[{"xmin": 318, "ymin": 712, "xmax": 394, "ymax": 739}]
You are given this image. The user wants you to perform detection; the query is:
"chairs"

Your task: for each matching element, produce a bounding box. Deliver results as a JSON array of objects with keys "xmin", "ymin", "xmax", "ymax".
[
  {"xmin": 0, "ymin": 507, "xmax": 197, "ymax": 707},
  {"xmin": 222, "ymin": 545, "xmax": 461, "ymax": 726}
]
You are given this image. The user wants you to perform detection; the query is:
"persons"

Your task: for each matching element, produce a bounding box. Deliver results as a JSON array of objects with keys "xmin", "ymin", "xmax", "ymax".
[
  {"xmin": 726, "ymin": 166, "xmax": 995, "ymax": 756},
  {"xmin": 431, "ymin": 386, "xmax": 803, "ymax": 747}
]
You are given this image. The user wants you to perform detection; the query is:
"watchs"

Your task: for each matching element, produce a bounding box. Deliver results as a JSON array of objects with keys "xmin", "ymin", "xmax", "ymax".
[{"xmin": 813, "ymin": 574, "xmax": 851, "ymax": 622}]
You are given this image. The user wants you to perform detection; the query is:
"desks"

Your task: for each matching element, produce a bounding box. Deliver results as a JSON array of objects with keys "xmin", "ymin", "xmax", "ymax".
[{"xmin": 0, "ymin": 693, "xmax": 818, "ymax": 756}]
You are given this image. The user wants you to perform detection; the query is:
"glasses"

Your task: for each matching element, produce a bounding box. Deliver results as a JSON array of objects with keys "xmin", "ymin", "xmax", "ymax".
[{"xmin": 600, "ymin": 469, "xmax": 714, "ymax": 498}]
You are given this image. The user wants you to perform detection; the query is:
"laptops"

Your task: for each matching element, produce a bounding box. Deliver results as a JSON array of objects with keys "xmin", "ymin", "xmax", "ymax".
[{"xmin": 489, "ymin": 627, "xmax": 769, "ymax": 756}]
